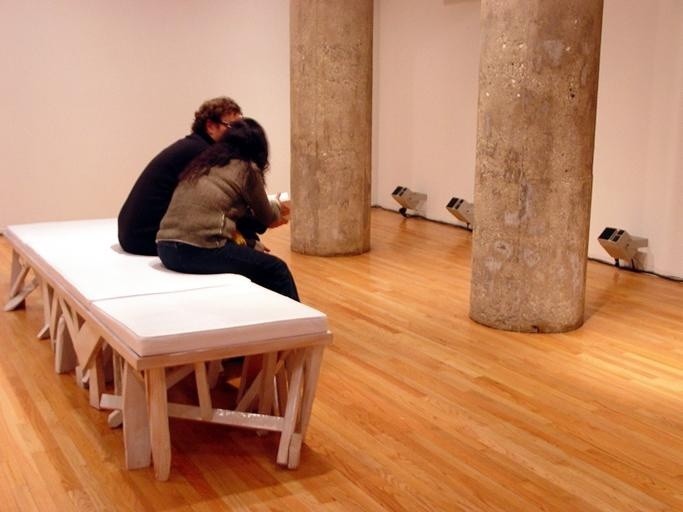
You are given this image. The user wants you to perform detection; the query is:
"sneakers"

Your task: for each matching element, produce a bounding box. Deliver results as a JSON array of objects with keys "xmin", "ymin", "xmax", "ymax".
[{"xmin": 207, "ymin": 361, "xmax": 223, "ymax": 388}]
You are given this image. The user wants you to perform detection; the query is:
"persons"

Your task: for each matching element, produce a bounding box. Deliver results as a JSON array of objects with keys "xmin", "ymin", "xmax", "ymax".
[
  {"xmin": 116, "ymin": 96, "xmax": 244, "ymax": 256},
  {"xmin": 156, "ymin": 117, "xmax": 299, "ymax": 365}
]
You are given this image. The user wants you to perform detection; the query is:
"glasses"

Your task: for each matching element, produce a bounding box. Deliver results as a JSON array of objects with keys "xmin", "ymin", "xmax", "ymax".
[{"xmin": 219, "ymin": 120, "xmax": 232, "ymax": 129}]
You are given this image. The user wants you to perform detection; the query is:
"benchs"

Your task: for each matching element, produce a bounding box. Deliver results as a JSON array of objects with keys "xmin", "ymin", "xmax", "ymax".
[{"xmin": 2, "ymin": 218, "xmax": 333, "ymax": 482}]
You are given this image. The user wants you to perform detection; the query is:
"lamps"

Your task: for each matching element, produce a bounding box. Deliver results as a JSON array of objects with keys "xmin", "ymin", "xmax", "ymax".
[
  {"xmin": 446, "ymin": 197, "xmax": 473, "ymax": 229},
  {"xmin": 392, "ymin": 186, "xmax": 420, "ymax": 218},
  {"xmin": 597, "ymin": 227, "xmax": 649, "ymax": 271}
]
